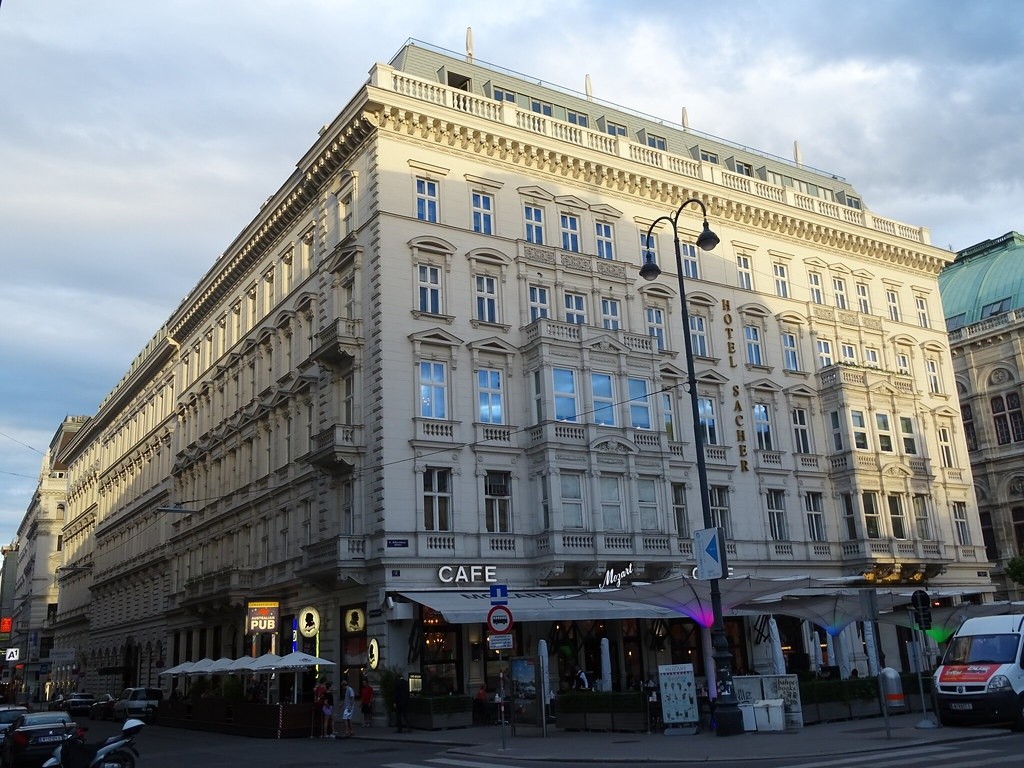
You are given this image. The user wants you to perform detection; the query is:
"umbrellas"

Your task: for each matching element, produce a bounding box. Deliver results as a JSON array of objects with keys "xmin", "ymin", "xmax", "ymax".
[
  {"xmin": 230, "ymin": 654, "xmax": 307, "ymax": 703},
  {"xmin": 182, "ymin": 659, "xmax": 233, "ymax": 694},
  {"xmin": 547, "ymin": 575, "xmax": 1024, "ymax": 703},
  {"xmin": 188, "ymin": 657, "xmax": 240, "ymax": 695},
  {"xmin": 257, "ymin": 652, "xmax": 336, "ymax": 704},
  {"xmin": 209, "ymin": 656, "xmax": 274, "ymax": 697},
  {"xmin": 158, "ymin": 662, "xmax": 204, "ymax": 695}
]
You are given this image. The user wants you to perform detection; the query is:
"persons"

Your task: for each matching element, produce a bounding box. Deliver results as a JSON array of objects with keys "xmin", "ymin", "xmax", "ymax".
[
  {"xmin": 478, "ymin": 683, "xmax": 489, "ymax": 723},
  {"xmin": 51, "ymin": 688, "xmax": 65, "ymax": 708},
  {"xmin": 72, "ymin": 687, "xmax": 85, "ymax": 699},
  {"xmin": 849, "ymin": 669, "xmax": 858, "ymax": 680},
  {"xmin": 631, "ymin": 679, "xmax": 660, "ymax": 697},
  {"xmin": 187, "ymin": 688, "xmax": 192, "ymax": 698},
  {"xmin": 315, "ymin": 677, "xmax": 338, "ymax": 737},
  {"xmin": 394, "ymin": 675, "xmax": 412, "ymax": 733},
  {"xmin": 695, "ymin": 680, "xmax": 707, "ymax": 696},
  {"xmin": 170, "ymin": 688, "xmax": 181, "ymax": 702},
  {"xmin": 283, "ymin": 699, "xmax": 291, "ymax": 704},
  {"xmin": 249, "ymin": 682, "xmax": 272, "ymax": 704},
  {"xmin": 339, "ymin": 681, "xmax": 354, "ymax": 736},
  {"xmin": 741, "ymin": 663, "xmax": 761, "ymax": 675},
  {"xmin": 572, "ymin": 665, "xmax": 589, "ymax": 689},
  {"xmin": 200, "ymin": 685, "xmax": 221, "ymax": 699},
  {"xmin": 359, "ymin": 680, "xmax": 373, "ymax": 727}
]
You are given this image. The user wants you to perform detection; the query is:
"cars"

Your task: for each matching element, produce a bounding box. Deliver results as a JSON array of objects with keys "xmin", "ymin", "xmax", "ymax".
[
  {"xmin": 87, "ymin": 694, "xmax": 118, "ymax": 721},
  {"xmin": 51, "ymin": 694, "xmax": 69, "ymax": 711},
  {"xmin": 0, "ymin": 705, "xmax": 31, "ymax": 748},
  {"xmin": 62, "ymin": 693, "xmax": 98, "ymax": 715},
  {"xmin": 0, "ymin": 710, "xmax": 89, "ymax": 768}
]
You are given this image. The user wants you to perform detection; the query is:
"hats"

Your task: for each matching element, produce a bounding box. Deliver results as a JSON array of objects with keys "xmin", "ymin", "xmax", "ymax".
[{"xmin": 340, "ymin": 680, "xmax": 346, "ymax": 685}]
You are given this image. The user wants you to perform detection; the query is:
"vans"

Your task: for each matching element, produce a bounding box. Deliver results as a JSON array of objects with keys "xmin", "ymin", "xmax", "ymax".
[
  {"xmin": 110, "ymin": 688, "xmax": 163, "ymax": 724},
  {"xmin": 929, "ymin": 614, "xmax": 1024, "ymax": 731}
]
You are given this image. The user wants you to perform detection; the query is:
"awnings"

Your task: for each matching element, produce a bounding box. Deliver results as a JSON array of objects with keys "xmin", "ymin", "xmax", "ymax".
[{"xmin": 398, "ymin": 589, "xmax": 895, "ymax": 624}]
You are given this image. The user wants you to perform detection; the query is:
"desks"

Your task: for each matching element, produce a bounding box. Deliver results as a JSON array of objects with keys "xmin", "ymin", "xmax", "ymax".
[{"xmin": 489, "ymin": 699, "xmax": 513, "ymax": 726}]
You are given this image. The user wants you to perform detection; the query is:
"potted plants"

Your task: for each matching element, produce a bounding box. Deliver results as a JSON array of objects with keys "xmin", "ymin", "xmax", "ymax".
[
  {"xmin": 800, "ymin": 670, "xmax": 935, "ymax": 724},
  {"xmin": 556, "ymin": 691, "xmax": 647, "ymax": 732},
  {"xmin": 378, "ymin": 667, "xmax": 403, "ymax": 726},
  {"xmin": 409, "ymin": 695, "xmax": 474, "ymax": 729}
]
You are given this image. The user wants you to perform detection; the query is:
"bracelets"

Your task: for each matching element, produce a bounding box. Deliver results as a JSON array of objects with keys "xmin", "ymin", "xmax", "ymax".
[{"xmin": 370, "ymin": 702, "xmax": 372, "ymax": 703}]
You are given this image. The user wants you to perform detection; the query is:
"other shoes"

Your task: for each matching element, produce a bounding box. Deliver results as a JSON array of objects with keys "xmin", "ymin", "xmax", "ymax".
[
  {"xmin": 362, "ymin": 724, "xmax": 366, "ymax": 728},
  {"xmin": 341, "ymin": 731, "xmax": 353, "ymax": 738},
  {"xmin": 394, "ymin": 730, "xmax": 402, "ymax": 733},
  {"xmin": 330, "ymin": 734, "xmax": 336, "ymax": 738},
  {"xmin": 324, "ymin": 734, "xmax": 329, "ymax": 738},
  {"xmin": 367, "ymin": 724, "xmax": 371, "ymax": 727}
]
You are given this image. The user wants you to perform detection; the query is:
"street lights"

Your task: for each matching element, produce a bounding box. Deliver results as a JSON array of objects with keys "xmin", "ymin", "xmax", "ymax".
[{"xmin": 638, "ymin": 196, "xmax": 748, "ymax": 738}]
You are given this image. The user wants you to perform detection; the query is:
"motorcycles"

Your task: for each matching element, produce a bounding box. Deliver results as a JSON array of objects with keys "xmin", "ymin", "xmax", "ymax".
[{"xmin": 40, "ymin": 717, "xmax": 147, "ymax": 768}]
